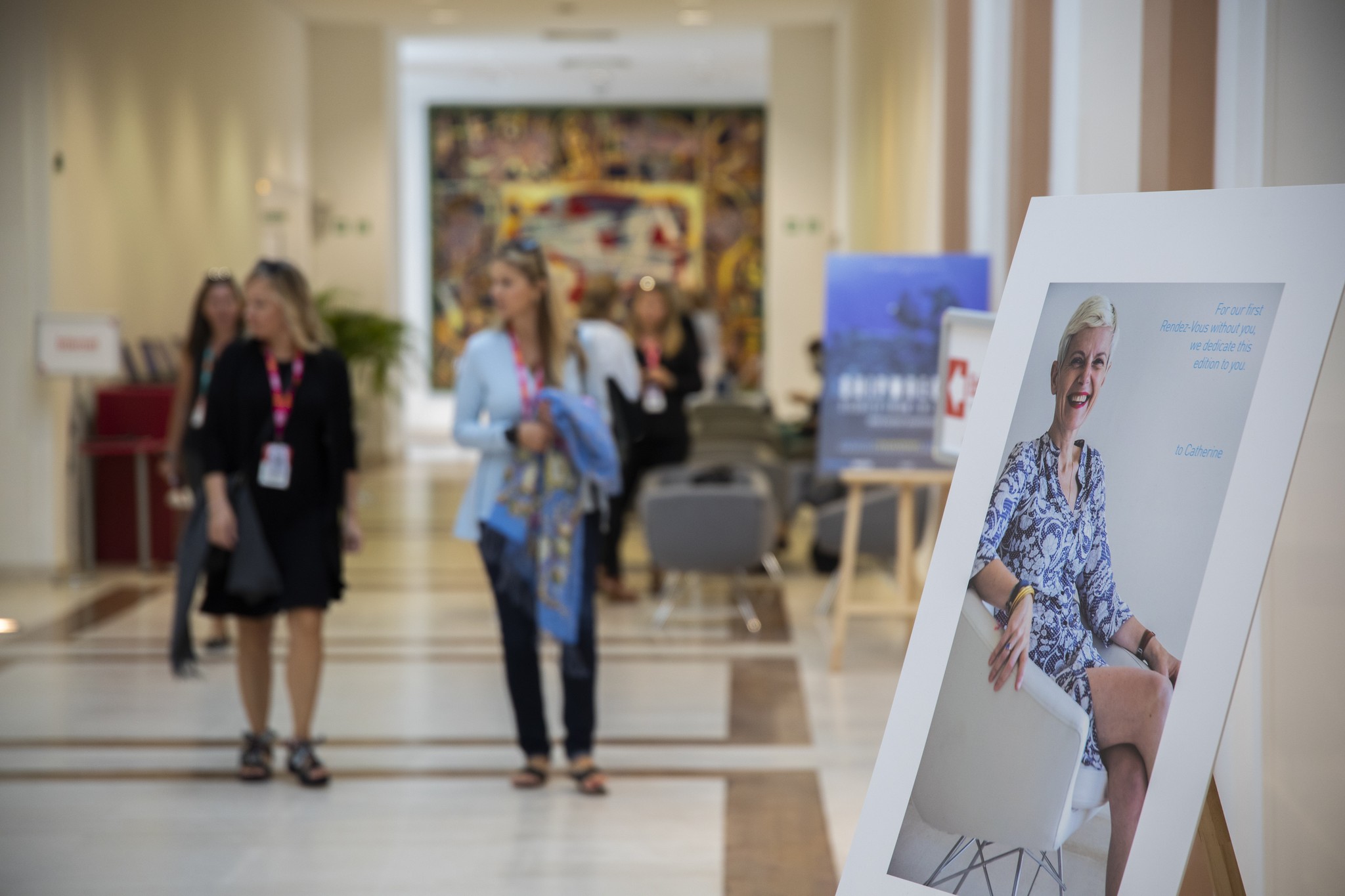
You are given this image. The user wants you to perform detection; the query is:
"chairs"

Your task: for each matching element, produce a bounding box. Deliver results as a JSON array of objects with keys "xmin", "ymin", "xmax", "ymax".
[
  {"xmin": 644, "ymin": 462, "xmax": 786, "ymax": 633},
  {"xmin": 907, "ymin": 592, "xmax": 1115, "ymax": 896}
]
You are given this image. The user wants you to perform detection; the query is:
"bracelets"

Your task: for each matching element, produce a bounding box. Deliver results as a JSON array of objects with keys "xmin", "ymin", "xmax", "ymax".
[
  {"xmin": 162, "ymin": 450, "xmax": 182, "ymax": 461},
  {"xmin": 1137, "ymin": 629, "xmax": 1156, "ymax": 660},
  {"xmin": 1004, "ymin": 579, "xmax": 1035, "ymax": 619}
]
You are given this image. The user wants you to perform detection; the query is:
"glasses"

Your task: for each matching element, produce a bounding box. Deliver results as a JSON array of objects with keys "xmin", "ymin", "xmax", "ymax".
[{"xmin": 506, "ymin": 235, "xmax": 539, "ymax": 254}]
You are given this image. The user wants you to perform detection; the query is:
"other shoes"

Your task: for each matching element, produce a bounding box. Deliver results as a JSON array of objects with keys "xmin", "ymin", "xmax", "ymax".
[{"xmin": 203, "ymin": 636, "xmax": 229, "ymax": 648}]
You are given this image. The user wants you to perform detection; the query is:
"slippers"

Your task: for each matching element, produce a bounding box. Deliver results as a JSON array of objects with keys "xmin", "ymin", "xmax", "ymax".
[
  {"xmin": 514, "ymin": 767, "xmax": 546, "ymax": 789},
  {"xmin": 572, "ymin": 765, "xmax": 605, "ymax": 795}
]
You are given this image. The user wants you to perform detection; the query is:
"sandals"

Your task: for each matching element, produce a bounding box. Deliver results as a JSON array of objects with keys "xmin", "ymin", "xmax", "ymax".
[
  {"xmin": 240, "ymin": 734, "xmax": 273, "ymax": 781},
  {"xmin": 285, "ymin": 736, "xmax": 331, "ymax": 786}
]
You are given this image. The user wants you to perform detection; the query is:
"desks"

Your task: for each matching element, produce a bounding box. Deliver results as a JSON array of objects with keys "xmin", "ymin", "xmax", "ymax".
[{"xmin": 827, "ymin": 469, "xmax": 956, "ymax": 672}]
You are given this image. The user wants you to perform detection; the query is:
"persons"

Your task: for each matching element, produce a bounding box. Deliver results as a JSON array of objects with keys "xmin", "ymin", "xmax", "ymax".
[
  {"xmin": 156, "ymin": 271, "xmax": 247, "ymax": 673},
  {"xmin": 965, "ymin": 298, "xmax": 1180, "ymax": 896},
  {"xmin": 444, "ymin": 235, "xmax": 617, "ymax": 796},
  {"xmin": 560, "ymin": 276, "xmax": 642, "ymax": 595},
  {"xmin": 769, "ymin": 340, "xmax": 858, "ymax": 549},
  {"xmin": 198, "ymin": 256, "xmax": 363, "ymax": 789},
  {"xmin": 602, "ymin": 278, "xmax": 707, "ymax": 599}
]
